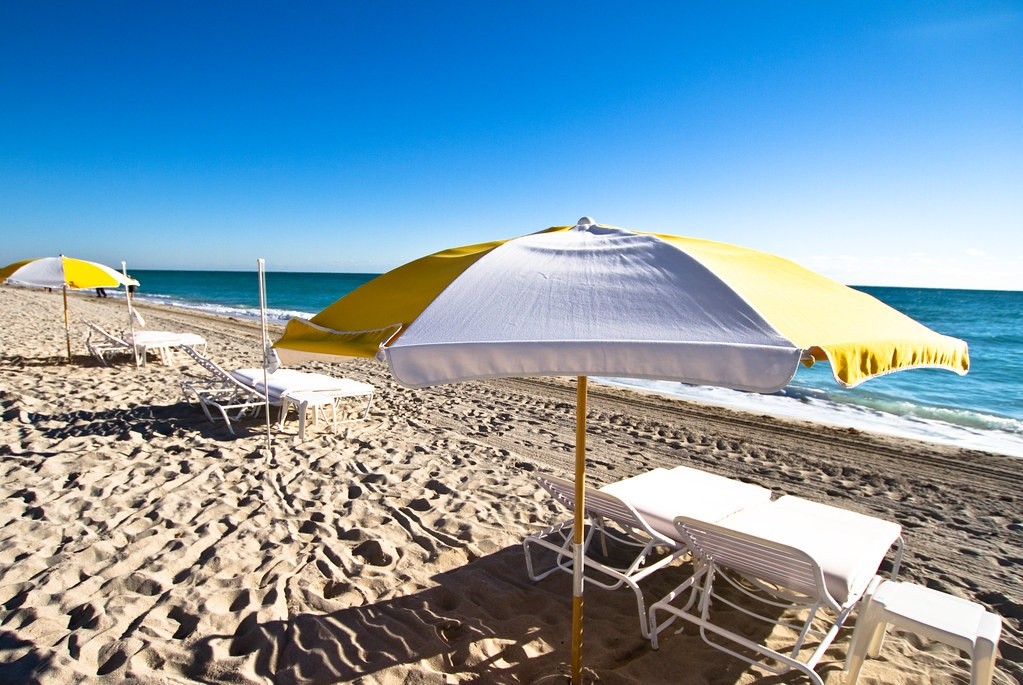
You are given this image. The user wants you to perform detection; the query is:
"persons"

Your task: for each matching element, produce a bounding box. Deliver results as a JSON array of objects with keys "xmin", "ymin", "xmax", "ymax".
[
  {"xmin": 127, "ymin": 275, "xmax": 136, "ymax": 301},
  {"xmin": 95, "ymin": 288, "xmax": 109, "ymax": 299}
]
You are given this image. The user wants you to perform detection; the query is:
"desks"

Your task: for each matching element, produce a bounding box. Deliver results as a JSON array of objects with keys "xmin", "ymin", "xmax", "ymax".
[
  {"xmin": 844, "ymin": 579, "xmax": 1003, "ymax": 685},
  {"xmin": 280, "ymin": 391, "xmax": 337, "ymax": 440},
  {"xmin": 130, "ymin": 339, "xmax": 173, "ymax": 369}
]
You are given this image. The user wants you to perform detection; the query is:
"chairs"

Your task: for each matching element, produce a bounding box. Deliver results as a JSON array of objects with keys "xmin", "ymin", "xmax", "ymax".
[
  {"xmin": 81, "ymin": 319, "xmax": 377, "ymax": 435},
  {"xmin": 523, "ymin": 464, "xmax": 905, "ymax": 685}
]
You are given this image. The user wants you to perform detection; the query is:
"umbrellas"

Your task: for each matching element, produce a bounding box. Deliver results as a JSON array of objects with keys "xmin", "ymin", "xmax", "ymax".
[
  {"xmin": 1, "ymin": 254, "xmax": 141, "ymax": 364},
  {"xmin": 271, "ymin": 217, "xmax": 968, "ymax": 684}
]
